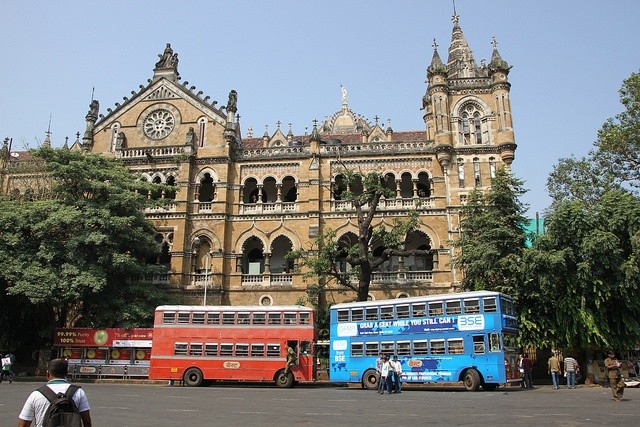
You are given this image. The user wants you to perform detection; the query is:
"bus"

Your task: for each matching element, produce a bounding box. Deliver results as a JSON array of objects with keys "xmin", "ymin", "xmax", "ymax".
[
  {"xmin": 149, "ymin": 305, "xmax": 316, "ymax": 387},
  {"xmin": 329, "ymin": 290, "xmax": 518, "ymax": 391}
]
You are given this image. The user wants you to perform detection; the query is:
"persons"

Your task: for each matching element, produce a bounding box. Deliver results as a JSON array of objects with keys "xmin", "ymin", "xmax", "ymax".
[
  {"xmin": 0, "ymin": 352, "xmax": 16, "ymax": 384},
  {"xmin": 546, "ymin": 353, "xmax": 562, "ymax": 389},
  {"xmin": 523, "ymin": 354, "xmax": 534, "ymax": 389},
  {"xmin": 379, "ymin": 356, "xmax": 391, "ymax": 395},
  {"xmin": 375, "ymin": 352, "xmax": 384, "ymax": 394},
  {"xmin": 283, "ymin": 345, "xmax": 299, "ymax": 374},
  {"xmin": 563, "ymin": 353, "xmax": 578, "ymax": 389},
  {"xmin": 517, "ymin": 353, "xmax": 532, "ymax": 389},
  {"xmin": 390, "ymin": 355, "xmax": 403, "ymax": 393},
  {"xmin": 18, "ymin": 358, "xmax": 92, "ymax": 426},
  {"xmin": 603, "ymin": 350, "xmax": 626, "ymax": 403},
  {"xmin": 385, "ymin": 354, "xmax": 394, "ymax": 394},
  {"xmin": 6, "ymin": 350, "xmax": 17, "ymax": 384}
]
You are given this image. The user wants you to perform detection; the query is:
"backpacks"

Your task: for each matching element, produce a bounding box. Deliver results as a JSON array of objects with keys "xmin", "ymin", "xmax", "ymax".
[{"xmin": 35, "ymin": 384, "xmax": 83, "ymax": 427}]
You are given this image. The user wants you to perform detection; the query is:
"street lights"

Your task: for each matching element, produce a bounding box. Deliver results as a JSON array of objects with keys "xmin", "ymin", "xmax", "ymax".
[{"xmin": 192, "ymin": 237, "xmax": 209, "ymax": 304}]
